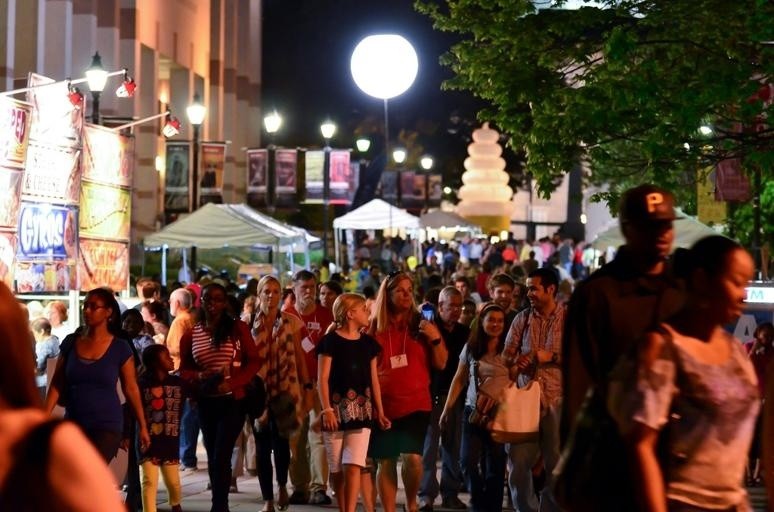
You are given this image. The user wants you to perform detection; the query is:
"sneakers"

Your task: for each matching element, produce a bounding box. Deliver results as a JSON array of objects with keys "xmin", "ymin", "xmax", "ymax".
[
  {"xmin": 179, "ymin": 462, "xmax": 198, "ymax": 473},
  {"xmin": 262, "ymin": 488, "xmax": 332, "ymax": 512},
  {"xmin": 207, "ymin": 478, "xmax": 237, "ymax": 494},
  {"xmin": 420, "ymin": 496, "xmax": 433, "ymax": 511},
  {"xmin": 442, "ymin": 492, "xmax": 467, "ymax": 509},
  {"xmin": 747, "ymin": 476, "xmax": 763, "ymax": 486}
]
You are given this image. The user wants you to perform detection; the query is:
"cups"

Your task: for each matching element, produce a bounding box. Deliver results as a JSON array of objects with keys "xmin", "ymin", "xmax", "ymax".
[{"xmin": 420, "ymin": 303, "xmax": 433, "ymax": 325}]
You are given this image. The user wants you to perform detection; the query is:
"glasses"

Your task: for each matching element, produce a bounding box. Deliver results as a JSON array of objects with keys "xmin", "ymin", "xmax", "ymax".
[{"xmin": 80, "ymin": 303, "xmax": 105, "ymax": 310}]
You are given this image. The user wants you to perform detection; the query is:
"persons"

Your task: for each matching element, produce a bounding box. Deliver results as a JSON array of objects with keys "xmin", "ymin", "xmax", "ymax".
[
  {"xmin": 1, "ymin": 227, "xmax": 774, "ymax": 512},
  {"xmin": 554, "ymin": 181, "xmax": 690, "ymax": 453}
]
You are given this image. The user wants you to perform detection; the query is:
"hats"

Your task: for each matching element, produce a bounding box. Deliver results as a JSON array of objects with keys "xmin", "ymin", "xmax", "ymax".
[{"xmin": 618, "ymin": 183, "xmax": 686, "ymax": 225}]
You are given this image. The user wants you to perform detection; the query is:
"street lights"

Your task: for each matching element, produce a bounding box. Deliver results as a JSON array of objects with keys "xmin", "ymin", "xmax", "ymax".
[
  {"xmin": 83, "ymin": 51, "xmax": 109, "ymax": 123},
  {"xmin": 355, "ymin": 137, "xmax": 372, "ymax": 200},
  {"xmin": 392, "ymin": 146, "xmax": 407, "ymax": 208},
  {"xmin": 186, "ymin": 95, "xmax": 208, "ymax": 210},
  {"xmin": 420, "ymin": 154, "xmax": 434, "ymax": 211},
  {"xmin": 319, "ymin": 115, "xmax": 336, "ymax": 254},
  {"xmin": 263, "ymin": 105, "xmax": 283, "ymax": 217}
]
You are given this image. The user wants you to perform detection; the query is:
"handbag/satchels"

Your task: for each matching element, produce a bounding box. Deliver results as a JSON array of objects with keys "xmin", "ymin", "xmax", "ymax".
[
  {"xmin": 550, "ymin": 380, "xmax": 711, "ymax": 512},
  {"xmin": 271, "ymin": 391, "xmax": 295, "ymax": 415},
  {"xmin": 489, "ymin": 376, "xmax": 541, "ymax": 444},
  {"xmin": 241, "ymin": 376, "xmax": 265, "ymax": 419}
]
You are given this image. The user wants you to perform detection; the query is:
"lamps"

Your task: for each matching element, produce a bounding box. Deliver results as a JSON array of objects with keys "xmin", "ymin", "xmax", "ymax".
[
  {"xmin": 113, "ymin": 109, "xmax": 180, "ymax": 138},
  {"xmin": 0, "ymin": 68, "xmax": 136, "ymax": 112}
]
